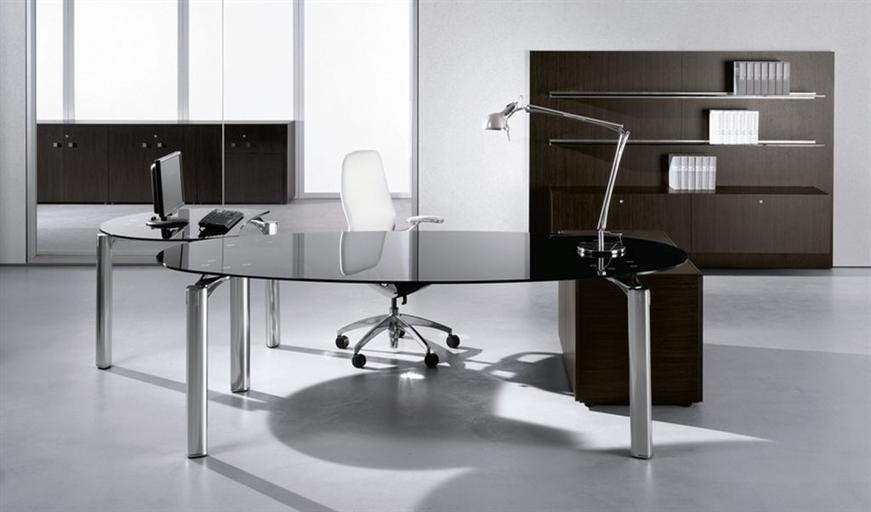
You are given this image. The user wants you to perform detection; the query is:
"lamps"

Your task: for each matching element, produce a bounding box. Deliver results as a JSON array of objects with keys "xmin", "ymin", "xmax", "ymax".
[{"xmin": 485, "ymin": 95, "xmax": 631, "ymax": 255}]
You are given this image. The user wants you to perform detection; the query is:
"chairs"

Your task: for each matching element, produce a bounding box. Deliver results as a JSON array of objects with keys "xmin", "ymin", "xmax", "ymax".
[{"xmin": 335, "ymin": 150, "xmax": 460, "ymax": 368}]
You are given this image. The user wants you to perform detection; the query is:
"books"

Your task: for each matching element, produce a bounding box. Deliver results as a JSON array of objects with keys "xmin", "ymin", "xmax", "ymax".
[
  {"xmin": 733, "ymin": 62, "xmax": 791, "ymax": 96},
  {"xmin": 668, "ymin": 154, "xmax": 717, "ymax": 192},
  {"xmin": 709, "ymin": 109, "xmax": 760, "ymax": 145}
]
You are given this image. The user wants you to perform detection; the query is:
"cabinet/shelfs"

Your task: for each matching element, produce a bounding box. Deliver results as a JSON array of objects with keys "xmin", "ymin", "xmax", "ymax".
[
  {"xmin": 548, "ymin": 186, "xmax": 692, "ymax": 269},
  {"xmin": 691, "ymin": 186, "xmax": 833, "ymax": 268},
  {"xmin": 36, "ymin": 120, "xmax": 108, "ymax": 203},
  {"xmin": 558, "ymin": 228, "xmax": 703, "ymax": 405},
  {"xmin": 546, "ymin": 91, "xmax": 827, "ymax": 148},
  {"xmin": 196, "ymin": 120, "xmax": 296, "ymax": 204},
  {"xmin": 111, "ymin": 120, "xmax": 198, "ymax": 204}
]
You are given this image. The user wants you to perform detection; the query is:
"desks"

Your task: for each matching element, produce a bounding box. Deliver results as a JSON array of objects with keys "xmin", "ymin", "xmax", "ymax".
[
  {"xmin": 156, "ymin": 231, "xmax": 690, "ymax": 460},
  {"xmin": 96, "ymin": 209, "xmax": 281, "ymax": 392}
]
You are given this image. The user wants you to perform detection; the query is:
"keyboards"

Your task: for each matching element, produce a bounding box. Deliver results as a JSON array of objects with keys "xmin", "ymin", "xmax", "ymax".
[{"xmin": 198, "ymin": 208, "xmax": 243, "ymax": 233}]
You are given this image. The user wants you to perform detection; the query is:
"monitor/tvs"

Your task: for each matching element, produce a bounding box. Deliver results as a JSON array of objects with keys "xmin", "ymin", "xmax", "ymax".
[{"xmin": 145, "ymin": 149, "xmax": 190, "ymax": 228}]
